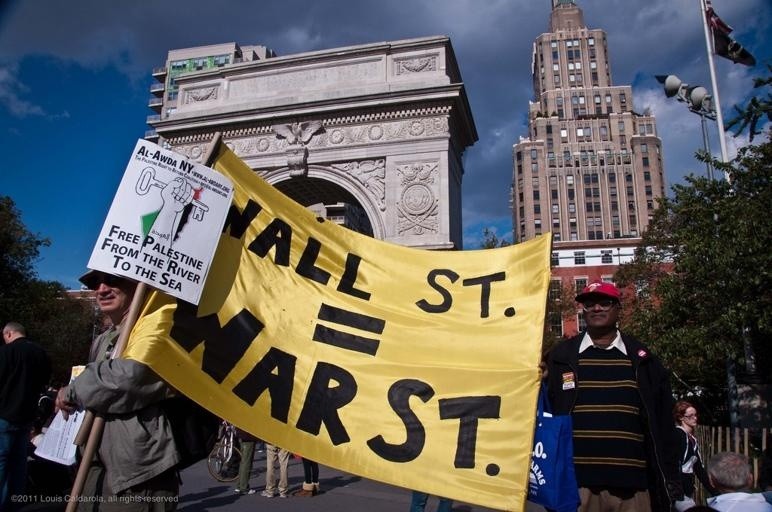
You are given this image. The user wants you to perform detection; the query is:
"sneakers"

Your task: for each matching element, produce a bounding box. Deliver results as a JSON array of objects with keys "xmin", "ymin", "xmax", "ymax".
[
  {"xmin": 233, "ymin": 488, "xmax": 241, "ymax": 496},
  {"xmin": 247, "ymin": 488, "xmax": 256, "ymax": 496},
  {"xmin": 260, "ymin": 490, "xmax": 275, "ymax": 498},
  {"xmin": 278, "ymin": 489, "xmax": 288, "ymax": 498}
]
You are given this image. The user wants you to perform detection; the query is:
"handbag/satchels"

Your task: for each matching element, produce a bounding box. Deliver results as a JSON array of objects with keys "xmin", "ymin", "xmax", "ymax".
[{"xmin": 527, "ymin": 381, "xmax": 583, "ymax": 512}]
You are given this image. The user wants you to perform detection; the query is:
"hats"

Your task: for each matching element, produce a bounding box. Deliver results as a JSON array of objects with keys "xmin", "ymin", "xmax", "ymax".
[
  {"xmin": 574, "ymin": 278, "xmax": 623, "ymax": 303},
  {"xmin": 77, "ymin": 269, "xmax": 139, "ymax": 286}
]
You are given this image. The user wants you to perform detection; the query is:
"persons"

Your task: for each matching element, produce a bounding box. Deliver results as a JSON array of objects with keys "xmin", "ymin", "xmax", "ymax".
[
  {"xmin": 410, "ymin": 488, "xmax": 454, "ymax": 511},
  {"xmin": 664, "ymin": 395, "xmax": 722, "ymax": 512},
  {"xmin": 539, "ymin": 279, "xmax": 676, "ymax": 512},
  {"xmin": 0, "ymin": 315, "xmax": 324, "ymax": 512},
  {"xmin": 51, "ymin": 266, "xmax": 185, "ymax": 510},
  {"xmin": 703, "ymin": 451, "xmax": 772, "ymax": 512},
  {"xmin": 757, "ymin": 449, "xmax": 772, "ymax": 505}
]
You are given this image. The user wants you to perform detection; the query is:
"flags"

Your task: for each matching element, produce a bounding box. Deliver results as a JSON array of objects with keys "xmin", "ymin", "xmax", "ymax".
[
  {"xmin": 705, "ymin": 0, "xmax": 735, "ymax": 37},
  {"xmin": 709, "ymin": 26, "xmax": 757, "ymax": 69}
]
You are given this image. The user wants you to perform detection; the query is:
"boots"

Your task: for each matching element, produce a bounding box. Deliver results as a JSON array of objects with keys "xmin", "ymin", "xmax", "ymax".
[{"xmin": 292, "ymin": 481, "xmax": 321, "ymax": 498}]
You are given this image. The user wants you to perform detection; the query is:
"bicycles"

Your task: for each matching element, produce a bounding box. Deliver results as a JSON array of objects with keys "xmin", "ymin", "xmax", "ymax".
[{"xmin": 206, "ymin": 418, "xmax": 242, "ymax": 483}]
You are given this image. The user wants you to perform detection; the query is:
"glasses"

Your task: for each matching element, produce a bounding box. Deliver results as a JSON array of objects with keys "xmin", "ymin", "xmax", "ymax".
[{"xmin": 581, "ymin": 300, "xmax": 615, "ymax": 312}]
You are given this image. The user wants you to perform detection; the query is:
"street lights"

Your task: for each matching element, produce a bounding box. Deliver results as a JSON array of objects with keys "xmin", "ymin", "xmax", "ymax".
[{"xmin": 656, "ymin": 72, "xmax": 743, "ymax": 444}]
